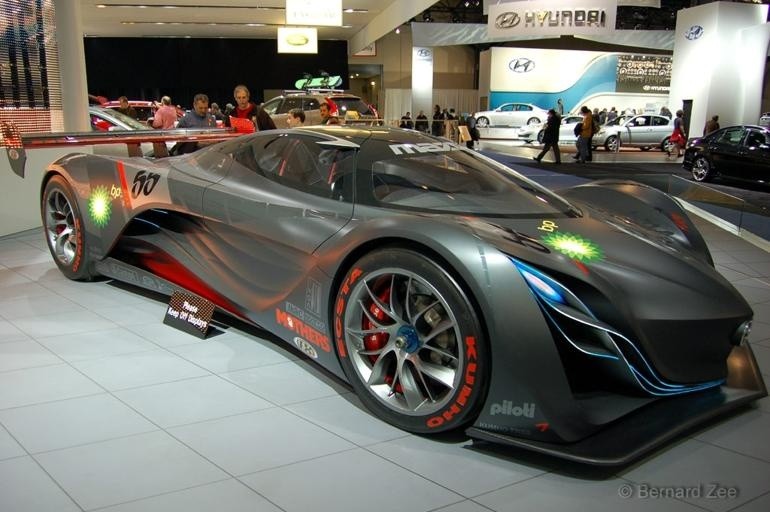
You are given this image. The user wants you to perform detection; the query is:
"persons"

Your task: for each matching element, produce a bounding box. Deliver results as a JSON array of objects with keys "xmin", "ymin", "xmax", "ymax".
[
  {"xmin": 532, "ymin": 108, "xmax": 562, "ymax": 162},
  {"xmin": 398, "ymin": 104, "xmax": 480, "ymax": 152},
  {"xmin": 557, "ymin": 98, "xmax": 566, "ymax": 116},
  {"xmin": 703, "ymin": 114, "xmax": 721, "ymax": 138},
  {"xmin": 573, "ymin": 102, "xmax": 673, "ymax": 163},
  {"xmin": 117, "ymin": 83, "xmax": 340, "ymax": 156},
  {"xmin": 667, "ymin": 110, "xmax": 688, "ymax": 158}
]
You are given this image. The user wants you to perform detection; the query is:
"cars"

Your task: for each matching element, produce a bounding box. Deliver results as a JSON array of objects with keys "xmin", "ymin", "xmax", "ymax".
[
  {"xmin": 758, "ymin": 117, "xmax": 770, "ymax": 126},
  {"xmin": 84, "ymin": 104, "xmax": 177, "ymax": 159},
  {"xmin": 517, "ymin": 115, "xmax": 584, "ymax": 145},
  {"xmin": 681, "ymin": 121, "xmax": 769, "ymax": 187},
  {"xmin": 586, "ymin": 114, "xmax": 676, "ymax": 152},
  {"xmin": 473, "ymin": 103, "xmax": 549, "ymax": 127}
]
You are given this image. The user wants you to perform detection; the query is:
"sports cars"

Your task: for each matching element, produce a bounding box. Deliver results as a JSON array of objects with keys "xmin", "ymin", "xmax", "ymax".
[{"xmin": 1, "ymin": 112, "xmax": 769, "ymax": 478}]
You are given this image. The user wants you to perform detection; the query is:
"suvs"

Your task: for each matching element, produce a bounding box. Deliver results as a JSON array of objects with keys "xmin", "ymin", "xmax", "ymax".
[
  {"xmin": 256, "ymin": 88, "xmax": 381, "ymax": 131},
  {"xmin": 99, "ymin": 99, "xmax": 226, "ymax": 129}
]
